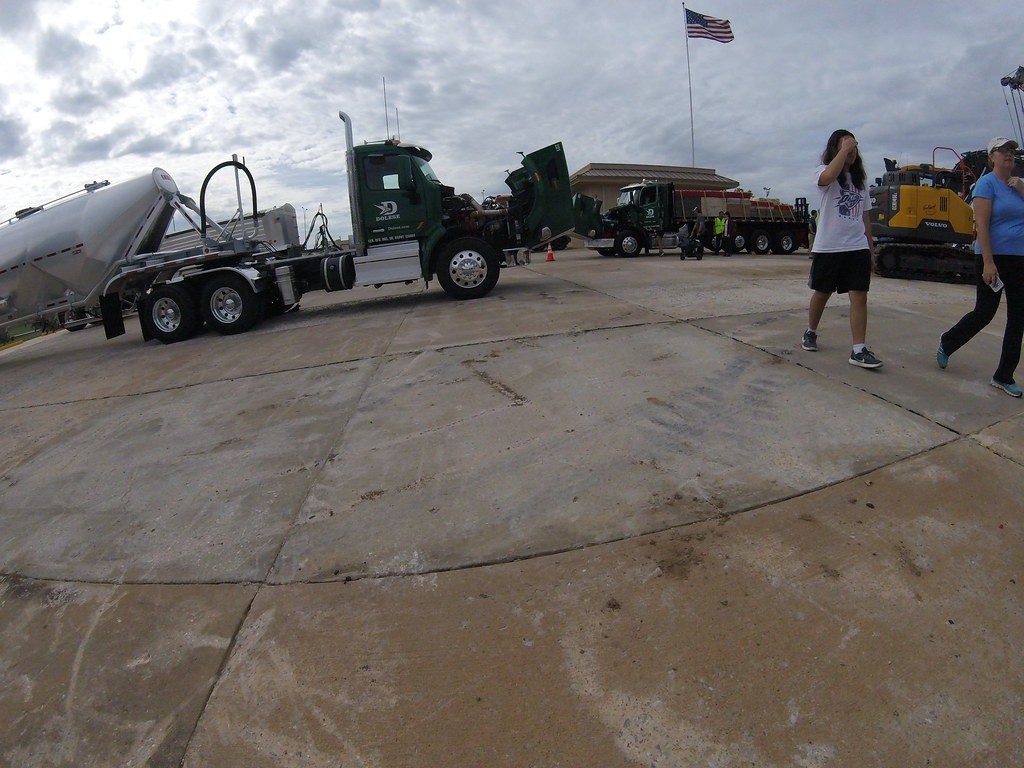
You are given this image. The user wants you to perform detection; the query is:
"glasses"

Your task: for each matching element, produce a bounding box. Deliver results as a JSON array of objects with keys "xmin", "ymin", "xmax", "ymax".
[{"xmin": 991, "ymin": 147, "xmax": 1016, "ymax": 155}]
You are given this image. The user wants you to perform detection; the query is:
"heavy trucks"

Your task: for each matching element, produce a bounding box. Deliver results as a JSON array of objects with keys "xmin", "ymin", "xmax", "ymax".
[{"xmin": 584, "ymin": 178, "xmax": 811, "ymax": 257}]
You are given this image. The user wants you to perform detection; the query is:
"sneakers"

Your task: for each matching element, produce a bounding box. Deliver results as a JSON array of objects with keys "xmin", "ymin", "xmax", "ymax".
[
  {"xmin": 935, "ymin": 331, "xmax": 949, "ymax": 368},
  {"xmin": 848, "ymin": 347, "xmax": 884, "ymax": 368},
  {"xmin": 990, "ymin": 376, "xmax": 1023, "ymax": 396},
  {"xmin": 800, "ymin": 328, "xmax": 819, "ymax": 351}
]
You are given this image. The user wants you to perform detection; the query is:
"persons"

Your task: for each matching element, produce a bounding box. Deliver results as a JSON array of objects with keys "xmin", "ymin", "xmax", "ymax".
[
  {"xmin": 802, "ymin": 128, "xmax": 885, "ymax": 367},
  {"xmin": 936, "ymin": 136, "xmax": 1024, "ymax": 397},
  {"xmin": 655, "ymin": 206, "xmax": 735, "ymax": 261},
  {"xmin": 808, "ymin": 208, "xmax": 818, "ymax": 259}
]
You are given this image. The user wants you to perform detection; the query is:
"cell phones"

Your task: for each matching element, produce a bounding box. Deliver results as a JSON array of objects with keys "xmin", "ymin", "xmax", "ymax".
[{"xmin": 981, "ymin": 274, "xmax": 1004, "ymax": 293}]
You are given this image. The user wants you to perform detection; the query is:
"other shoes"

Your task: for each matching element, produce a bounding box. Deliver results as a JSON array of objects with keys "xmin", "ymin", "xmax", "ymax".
[
  {"xmin": 726, "ymin": 254, "xmax": 731, "ymax": 256},
  {"xmin": 711, "ymin": 252, "xmax": 719, "ymax": 255},
  {"xmin": 660, "ymin": 252, "xmax": 665, "ymax": 256}
]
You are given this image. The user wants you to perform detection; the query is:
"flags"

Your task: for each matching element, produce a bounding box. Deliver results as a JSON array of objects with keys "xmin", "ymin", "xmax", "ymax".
[{"xmin": 684, "ymin": 7, "xmax": 735, "ymax": 44}]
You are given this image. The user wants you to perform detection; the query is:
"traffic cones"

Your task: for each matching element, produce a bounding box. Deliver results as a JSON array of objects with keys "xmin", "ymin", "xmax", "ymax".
[{"xmin": 545, "ymin": 242, "xmax": 556, "ymax": 262}]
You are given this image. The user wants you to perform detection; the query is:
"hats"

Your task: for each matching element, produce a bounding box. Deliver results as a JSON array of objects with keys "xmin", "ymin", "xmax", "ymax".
[
  {"xmin": 987, "ymin": 136, "xmax": 1019, "ymax": 155},
  {"xmin": 692, "ymin": 207, "xmax": 701, "ymax": 213}
]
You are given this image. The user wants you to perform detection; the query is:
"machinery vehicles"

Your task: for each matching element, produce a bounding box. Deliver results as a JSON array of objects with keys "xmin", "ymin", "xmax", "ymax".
[
  {"xmin": 0, "ymin": 106, "xmax": 576, "ymax": 345},
  {"xmin": 866, "ymin": 146, "xmax": 980, "ymax": 285}
]
